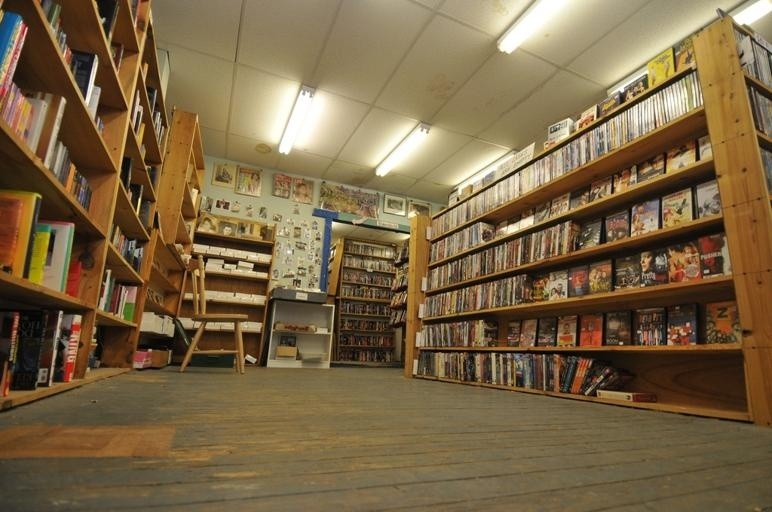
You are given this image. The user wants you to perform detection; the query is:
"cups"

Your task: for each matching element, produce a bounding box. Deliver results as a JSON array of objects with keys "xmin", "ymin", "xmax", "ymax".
[{"xmin": 319, "ymin": 353, "xmax": 328, "ymax": 361}]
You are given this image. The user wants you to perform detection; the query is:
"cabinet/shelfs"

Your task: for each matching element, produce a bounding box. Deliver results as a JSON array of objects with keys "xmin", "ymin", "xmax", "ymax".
[
  {"xmin": 176, "ymin": 200, "xmax": 280, "ymax": 375},
  {"xmin": 130, "ymin": 225, "xmax": 190, "ymax": 370},
  {"xmin": 409, "ymin": 19, "xmax": 771, "ymax": 427},
  {"xmin": 328, "ymin": 234, "xmax": 409, "ymax": 369},
  {"xmin": 154, "ymin": 104, "xmax": 212, "ymax": 269},
  {"xmin": 263, "ymin": 284, "xmax": 337, "ymax": 373},
  {"xmin": 0, "ymin": 1, "xmax": 171, "ymax": 409}
]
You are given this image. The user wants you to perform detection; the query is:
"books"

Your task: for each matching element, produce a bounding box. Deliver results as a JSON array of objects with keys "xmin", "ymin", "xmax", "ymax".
[
  {"xmin": 36, "ymin": 0, "xmax": 140, "ymax": 136},
  {"xmin": 177, "ymin": 245, "xmax": 271, "ymax": 334},
  {"xmin": 197, "ymin": 213, "xmax": 270, "ymax": 242},
  {"xmin": 107, "ymin": 224, "xmax": 143, "ymax": 274},
  {"xmin": 0, "ymin": 1, "xmax": 93, "ymax": 212},
  {"xmin": 1, "ymin": 308, "xmax": 83, "ymax": 398},
  {"xmin": 339, "ymin": 22, "xmax": 771, "ymax": 401},
  {"xmin": 130, "ymin": 62, "xmax": 165, "ymax": 193},
  {"xmin": 96, "ymin": 269, "xmax": 138, "ymax": 322},
  {"xmin": 135, "ymin": 311, "xmax": 176, "ymax": 352},
  {"xmin": 119, "ymin": 156, "xmax": 151, "ymax": 236},
  {"xmin": 1, "ymin": 188, "xmax": 82, "ymax": 297}
]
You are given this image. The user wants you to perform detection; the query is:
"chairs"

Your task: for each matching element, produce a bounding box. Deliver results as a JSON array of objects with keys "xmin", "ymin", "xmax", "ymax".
[{"xmin": 179, "ymin": 255, "xmax": 251, "ymax": 373}]
